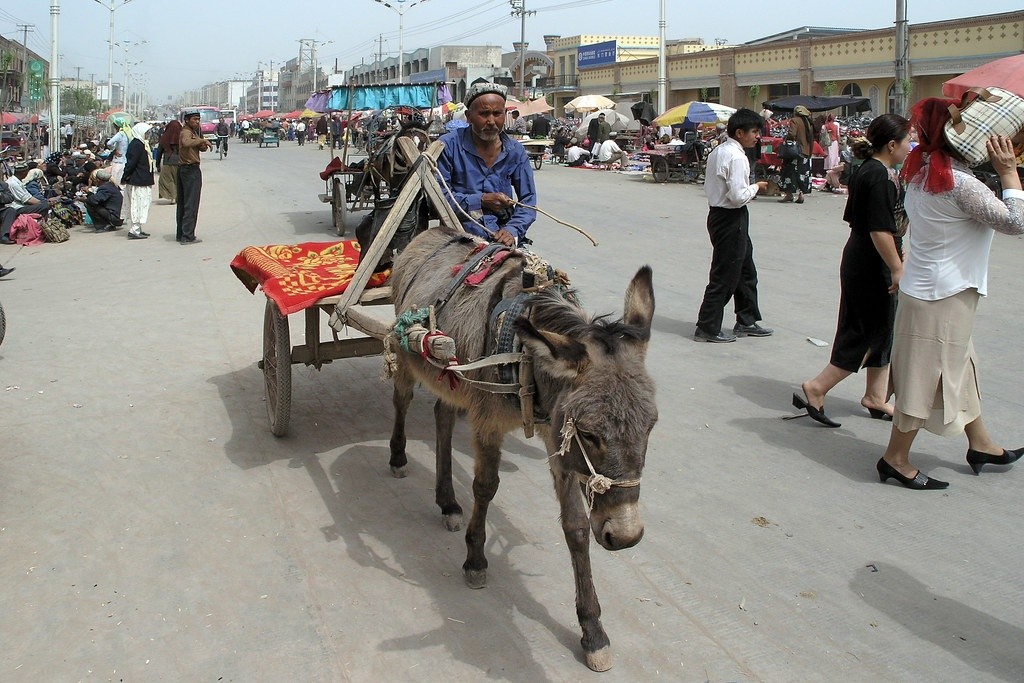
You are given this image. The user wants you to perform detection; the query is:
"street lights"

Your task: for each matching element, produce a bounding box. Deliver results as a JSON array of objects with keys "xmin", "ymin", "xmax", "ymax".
[
  {"xmin": 259, "ymin": 61, "xmax": 287, "ymax": 111},
  {"xmin": 236, "ymin": 72, "xmax": 255, "ymax": 114},
  {"xmin": 106, "ymin": 39, "xmax": 150, "ymax": 119},
  {"xmin": 295, "ymin": 39, "xmax": 335, "ymax": 92},
  {"xmin": 376, "ymin": 0, "xmax": 425, "ymax": 82}
]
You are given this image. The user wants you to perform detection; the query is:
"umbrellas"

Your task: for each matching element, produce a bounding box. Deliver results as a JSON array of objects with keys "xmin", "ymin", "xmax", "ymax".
[
  {"xmin": 652, "ymin": 100, "xmax": 739, "ymax": 128},
  {"xmin": 504, "ymin": 96, "xmax": 554, "ymax": 117},
  {"xmin": 254, "ymin": 109, "xmax": 326, "ymax": 117},
  {"xmin": 564, "ymin": 94, "xmax": 617, "ymax": 113},
  {"xmin": 576, "ymin": 109, "xmax": 629, "ymax": 134},
  {"xmin": 942, "ymin": 54, "xmax": 1024, "ymax": 101}
]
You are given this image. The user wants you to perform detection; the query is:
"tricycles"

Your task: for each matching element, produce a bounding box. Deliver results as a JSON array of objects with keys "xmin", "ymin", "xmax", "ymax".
[
  {"xmin": 648, "ymin": 137, "xmax": 717, "ymax": 183},
  {"xmin": 258, "ymin": 129, "xmax": 280, "ymax": 148},
  {"xmin": 507, "ymin": 134, "xmax": 545, "ymax": 170},
  {"xmin": 612, "ymin": 121, "xmax": 642, "ymax": 151}
]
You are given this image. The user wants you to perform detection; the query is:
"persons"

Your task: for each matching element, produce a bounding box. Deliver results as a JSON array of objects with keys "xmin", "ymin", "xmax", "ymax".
[
  {"xmin": 123, "ymin": 123, "xmax": 154, "ymax": 240},
  {"xmin": 218, "ymin": 117, "xmax": 361, "ymax": 157},
  {"xmin": 0, "ymin": 264, "xmax": 15, "ymax": 277},
  {"xmin": 510, "ymin": 111, "xmax": 629, "ymax": 169},
  {"xmin": 0, "ymin": 122, "xmax": 167, "ymax": 245},
  {"xmin": 759, "ymin": 106, "xmax": 841, "ymax": 205},
  {"xmin": 157, "ymin": 120, "xmax": 182, "ymax": 203},
  {"xmin": 85, "ymin": 170, "xmax": 123, "ymax": 230},
  {"xmin": 695, "ymin": 108, "xmax": 773, "ymax": 344},
  {"xmin": 433, "ymin": 82, "xmax": 536, "ymax": 249},
  {"xmin": 791, "ymin": 114, "xmax": 907, "ymax": 428},
  {"xmin": 876, "ymin": 97, "xmax": 1024, "ymax": 491},
  {"xmin": 176, "ymin": 109, "xmax": 213, "ymax": 245}
]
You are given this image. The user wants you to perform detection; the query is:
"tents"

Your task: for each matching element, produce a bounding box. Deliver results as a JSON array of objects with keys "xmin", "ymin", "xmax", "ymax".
[{"xmin": 762, "ymin": 94, "xmax": 871, "ymax": 113}]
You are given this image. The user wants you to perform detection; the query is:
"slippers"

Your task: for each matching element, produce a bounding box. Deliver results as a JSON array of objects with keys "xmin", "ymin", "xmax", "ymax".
[
  {"xmin": 868, "ymin": 407, "xmax": 895, "ymax": 420},
  {"xmin": 792, "ymin": 392, "xmax": 841, "ymax": 427}
]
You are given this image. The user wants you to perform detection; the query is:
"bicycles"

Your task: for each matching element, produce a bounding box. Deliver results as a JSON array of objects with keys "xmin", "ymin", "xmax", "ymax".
[
  {"xmin": 353, "ymin": 132, "xmax": 377, "ymax": 155},
  {"xmin": 0, "ymin": 144, "xmax": 11, "ymax": 178},
  {"xmin": 215, "ymin": 134, "xmax": 230, "ymax": 161}
]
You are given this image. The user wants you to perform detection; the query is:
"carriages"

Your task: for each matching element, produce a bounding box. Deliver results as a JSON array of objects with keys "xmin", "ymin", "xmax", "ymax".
[{"xmin": 313, "ymin": 81, "xmax": 444, "ymax": 236}]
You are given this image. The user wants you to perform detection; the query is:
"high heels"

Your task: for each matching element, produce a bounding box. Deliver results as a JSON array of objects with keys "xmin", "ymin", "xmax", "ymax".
[
  {"xmin": 876, "ymin": 457, "xmax": 949, "ymax": 490},
  {"xmin": 966, "ymin": 448, "xmax": 1024, "ymax": 476}
]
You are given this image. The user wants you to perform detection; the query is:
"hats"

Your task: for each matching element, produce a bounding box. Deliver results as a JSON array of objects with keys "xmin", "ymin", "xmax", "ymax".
[
  {"xmin": 716, "ymin": 123, "xmax": 726, "ymax": 130},
  {"xmin": 185, "ymin": 109, "xmax": 200, "ymax": 115},
  {"xmin": 96, "ymin": 170, "xmax": 112, "ymax": 180},
  {"xmin": 600, "ymin": 115, "xmax": 617, "ymax": 136},
  {"xmin": 14, "ymin": 160, "xmax": 28, "ymax": 171},
  {"xmin": 557, "ymin": 127, "xmax": 577, "ymax": 144},
  {"xmin": 464, "ymin": 83, "xmax": 508, "ymax": 109},
  {"xmin": 71, "ymin": 144, "xmax": 91, "ymax": 159}
]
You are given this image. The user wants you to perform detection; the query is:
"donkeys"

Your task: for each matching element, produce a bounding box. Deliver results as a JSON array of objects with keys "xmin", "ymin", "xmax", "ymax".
[
  {"xmin": 389, "ymin": 225, "xmax": 660, "ymax": 672},
  {"xmin": 373, "ymin": 116, "xmax": 435, "ymax": 207}
]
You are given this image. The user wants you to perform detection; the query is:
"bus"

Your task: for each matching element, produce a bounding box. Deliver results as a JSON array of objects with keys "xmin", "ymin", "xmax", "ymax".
[
  {"xmin": 220, "ymin": 110, "xmax": 236, "ymax": 126},
  {"xmin": 179, "ymin": 104, "xmax": 222, "ymax": 140}
]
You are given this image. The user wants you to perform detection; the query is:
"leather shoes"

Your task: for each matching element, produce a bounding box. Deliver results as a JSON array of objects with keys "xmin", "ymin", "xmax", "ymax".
[
  {"xmin": 733, "ymin": 323, "xmax": 773, "ymax": 337},
  {"xmin": 694, "ymin": 327, "xmax": 737, "ymax": 342}
]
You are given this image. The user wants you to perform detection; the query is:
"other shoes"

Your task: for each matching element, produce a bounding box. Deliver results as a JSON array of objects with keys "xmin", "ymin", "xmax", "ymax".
[
  {"xmin": 128, "ymin": 231, "xmax": 150, "ymax": 238},
  {"xmin": 1, "ymin": 238, "xmax": 15, "ymax": 243},
  {"xmin": 0, "ymin": 267, "xmax": 15, "ymax": 277},
  {"xmin": 95, "ymin": 225, "xmax": 116, "ymax": 233},
  {"xmin": 319, "ymin": 146, "xmax": 342, "ymax": 150},
  {"xmin": 176, "ymin": 236, "xmax": 202, "ymax": 245},
  {"xmin": 216, "ymin": 150, "xmax": 219, "ymax": 153},
  {"xmin": 777, "ymin": 194, "xmax": 804, "ymax": 203},
  {"xmin": 171, "ymin": 199, "xmax": 176, "ymax": 204},
  {"xmin": 224, "ymin": 153, "xmax": 227, "ymax": 157}
]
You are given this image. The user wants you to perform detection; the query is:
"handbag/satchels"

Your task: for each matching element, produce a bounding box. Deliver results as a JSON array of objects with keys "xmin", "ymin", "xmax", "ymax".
[
  {"xmin": 944, "ymin": 86, "xmax": 1024, "ymax": 168},
  {"xmin": 776, "ymin": 135, "xmax": 805, "ymax": 159},
  {"xmin": 591, "ymin": 142, "xmax": 601, "ymax": 156},
  {"xmin": 819, "ymin": 125, "xmax": 831, "ymax": 147},
  {"xmin": 41, "ymin": 217, "xmax": 70, "ymax": 243}
]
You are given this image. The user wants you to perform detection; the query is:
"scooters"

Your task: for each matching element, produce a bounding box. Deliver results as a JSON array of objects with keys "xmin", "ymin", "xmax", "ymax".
[
  {"xmin": 554, "ymin": 117, "xmax": 581, "ymax": 135},
  {"xmin": 770, "ymin": 113, "xmax": 874, "ymax": 137}
]
